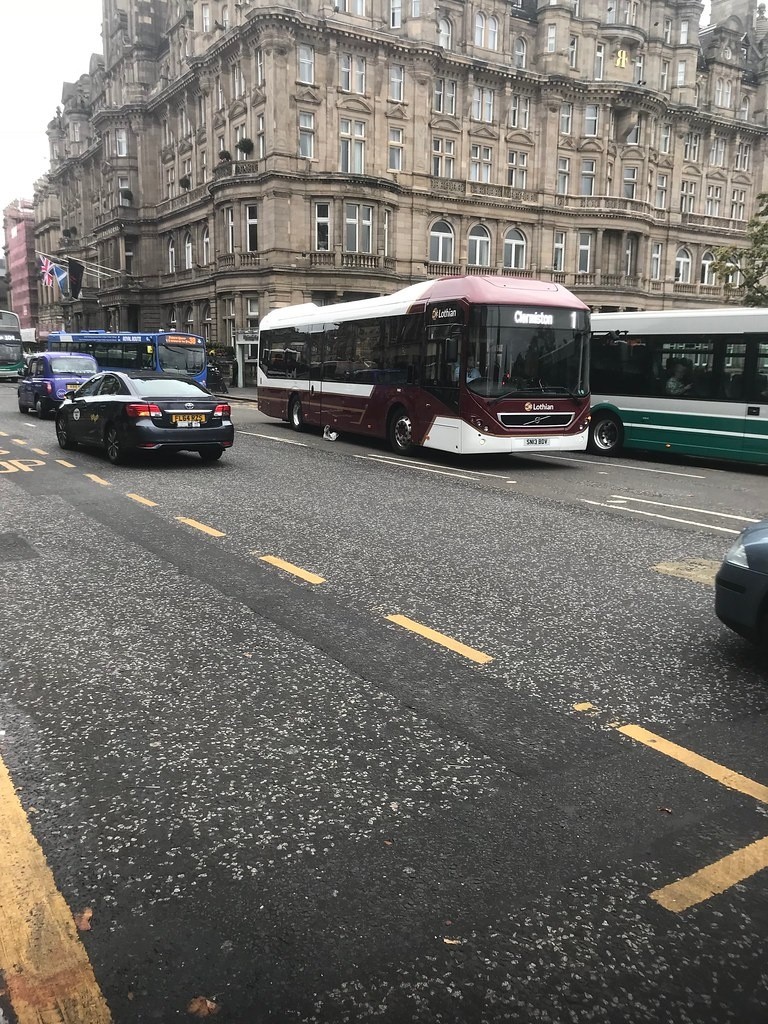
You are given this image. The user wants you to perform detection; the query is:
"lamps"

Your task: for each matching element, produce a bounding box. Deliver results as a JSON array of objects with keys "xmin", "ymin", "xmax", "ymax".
[
  {"xmin": 608, "ymin": 6, "xmax": 613, "ymax": 12},
  {"xmin": 654, "ymin": 21, "xmax": 659, "ymax": 27},
  {"xmin": 637, "ymin": 80, "xmax": 646, "ymax": 87},
  {"xmin": 334, "ymin": 7, "xmax": 340, "ymax": 13}
]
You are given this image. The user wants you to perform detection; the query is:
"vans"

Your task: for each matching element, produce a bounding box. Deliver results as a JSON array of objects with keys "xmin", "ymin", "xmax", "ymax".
[{"xmin": 18, "ymin": 353, "xmax": 99, "ymax": 419}]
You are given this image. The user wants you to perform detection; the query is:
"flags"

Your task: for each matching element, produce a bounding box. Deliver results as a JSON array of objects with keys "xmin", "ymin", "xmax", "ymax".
[
  {"xmin": 53, "ymin": 264, "xmax": 68, "ymax": 297},
  {"xmin": 67, "ymin": 258, "xmax": 85, "ymax": 301},
  {"xmin": 39, "ymin": 255, "xmax": 57, "ymax": 288}
]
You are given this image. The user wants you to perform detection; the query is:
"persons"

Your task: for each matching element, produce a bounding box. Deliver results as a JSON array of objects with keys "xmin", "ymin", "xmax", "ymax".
[
  {"xmin": 453, "ymin": 354, "xmax": 481, "ymax": 384},
  {"xmin": 665, "ymin": 362, "xmax": 694, "ymax": 397}
]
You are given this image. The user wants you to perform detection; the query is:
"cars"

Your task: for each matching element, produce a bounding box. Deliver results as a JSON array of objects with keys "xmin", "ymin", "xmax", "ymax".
[{"xmin": 56, "ymin": 370, "xmax": 236, "ymax": 464}]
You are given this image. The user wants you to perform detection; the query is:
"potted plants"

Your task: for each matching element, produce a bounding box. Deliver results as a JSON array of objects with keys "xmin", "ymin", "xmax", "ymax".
[{"xmin": 122, "ymin": 190, "xmax": 134, "ymax": 207}]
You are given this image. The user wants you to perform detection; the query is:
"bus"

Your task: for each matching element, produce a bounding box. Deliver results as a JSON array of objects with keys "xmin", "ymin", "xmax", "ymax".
[
  {"xmin": 254, "ymin": 274, "xmax": 633, "ymax": 459},
  {"xmin": 0, "ymin": 310, "xmax": 23, "ymax": 382},
  {"xmin": 48, "ymin": 329, "xmax": 216, "ymax": 390},
  {"xmin": 566, "ymin": 305, "xmax": 768, "ymax": 467}
]
